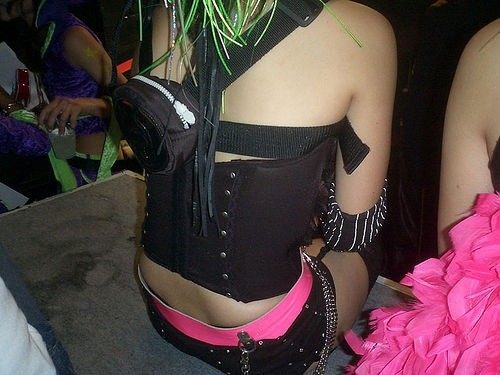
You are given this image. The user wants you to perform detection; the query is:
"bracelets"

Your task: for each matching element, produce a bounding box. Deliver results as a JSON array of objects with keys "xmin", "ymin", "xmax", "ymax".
[{"xmin": 4, "ymin": 100, "xmax": 21, "ymax": 114}]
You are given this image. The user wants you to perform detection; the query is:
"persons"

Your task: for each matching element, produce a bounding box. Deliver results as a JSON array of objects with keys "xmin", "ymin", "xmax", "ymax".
[
  {"xmin": 434, "ymin": 19, "xmax": 499, "ymax": 258},
  {"xmin": 119, "ymin": 0, "xmax": 395, "ymax": 374},
  {"xmin": 0, "ymin": 37, "xmax": 63, "ymax": 216},
  {"xmin": 1, "ymin": 0, "xmax": 129, "ymax": 195},
  {"xmin": 0, "ymin": 248, "xmax": 79, "ymax": 375}
]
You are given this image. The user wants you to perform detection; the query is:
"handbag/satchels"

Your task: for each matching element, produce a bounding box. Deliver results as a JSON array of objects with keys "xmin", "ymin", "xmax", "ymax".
[{"xmin": 111, "ymin": 73, "xmax": 206, "ymax": 173}]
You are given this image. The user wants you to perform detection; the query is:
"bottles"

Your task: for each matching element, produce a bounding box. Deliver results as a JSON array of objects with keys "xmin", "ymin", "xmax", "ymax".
[{"xmin": 49, "ymin": 114, "xmax": 77, "ymax": 159}]
